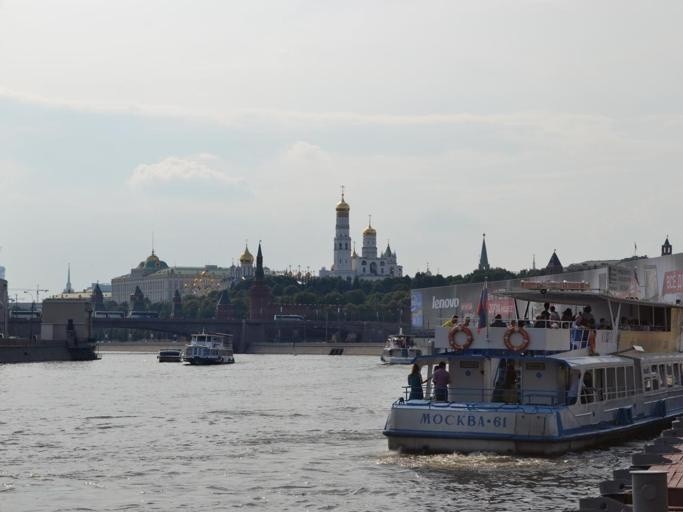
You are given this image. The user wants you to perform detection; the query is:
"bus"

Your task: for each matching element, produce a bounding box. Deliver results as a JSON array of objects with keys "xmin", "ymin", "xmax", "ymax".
[
  {"xmin": 8, "ymin": 310, "xmax": 40, "ymax": 319},
  {"xmin": 273, "ymin": 314, "xmax": 304, "ymax": 320},
  {"xmin": 129, "ymin": 311, "xmax": 158, "ymax": 319},
  {"xmin": 94, "ymin": 310, "xmax": 125, "ymax": 320}
]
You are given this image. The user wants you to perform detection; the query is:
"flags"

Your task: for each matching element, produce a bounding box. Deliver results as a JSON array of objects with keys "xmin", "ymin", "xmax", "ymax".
[{"xmin": 474, "ymin": 288, "xmax": 488, "ymax": 335}]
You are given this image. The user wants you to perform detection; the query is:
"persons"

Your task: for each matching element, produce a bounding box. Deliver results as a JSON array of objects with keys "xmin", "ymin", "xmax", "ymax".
[
  {"xmin": 490, "ymin": 358, "xmax": 506, "ymax": 402},
  {"xmin": 406, "ymin": 362, "xmax": 423, "ymax": 401},
  {"xmin": 430, "ymin": 360, "xmax": 449, "ymax": 402},
  {"xmin": 489, "ymin": 313, "xmax": 507, "ymax": 327},
  {"xmin": 456, "ymin": 315, "xmax": 470, "ymax": 327},
  {"xmin": 566, "ymin": 371, "xmax": 591, "ymax": 405},
  {"xmin": 491, "ymin": 358, "xmax": 516, "ymax": 403},
  {"xmin": 432, "ymin": 365, "xmax": 439, "ymax": 399},
  {"xmin": 441, "ymin": 314, "xmax": 458, "ymax": 328},
  {"xmin": 511, "ymin": 302, "xmax": 671, "ymax": 331}
]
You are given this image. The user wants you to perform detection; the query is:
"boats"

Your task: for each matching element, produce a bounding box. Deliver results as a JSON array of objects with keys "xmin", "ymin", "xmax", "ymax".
[
  {"xmin": 378, "ymin": 331, "xmax": 420, "ymax": 365},
  {"xmin": 183, "ymin": 330, "xmax": 236, "ymax": 368},
  {"xmin": 380, "ymin": 278, "xmax": 682, "ymax": 462},
  {"xmin": 156, "ymin": 348, "xmax": 182, "ymax": 363}
]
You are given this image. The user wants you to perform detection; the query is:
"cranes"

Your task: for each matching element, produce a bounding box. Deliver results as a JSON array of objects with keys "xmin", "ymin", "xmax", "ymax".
[{"xmin": 8, "ymin": 283, "xmax": 48, "ymax": 302}]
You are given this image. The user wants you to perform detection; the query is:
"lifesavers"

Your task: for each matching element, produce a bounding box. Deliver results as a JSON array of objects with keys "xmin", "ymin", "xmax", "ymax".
[
  {"xmin": 591, "ymin": 335, "xmax": 597, "ymax": 352},
  {"xmin": 449, "ymin": 326, "xmax": 473, "ymax": 350},
  {"xmin": 504, "ymin": 327, "xmax": 529, "ymax": 351}
]
